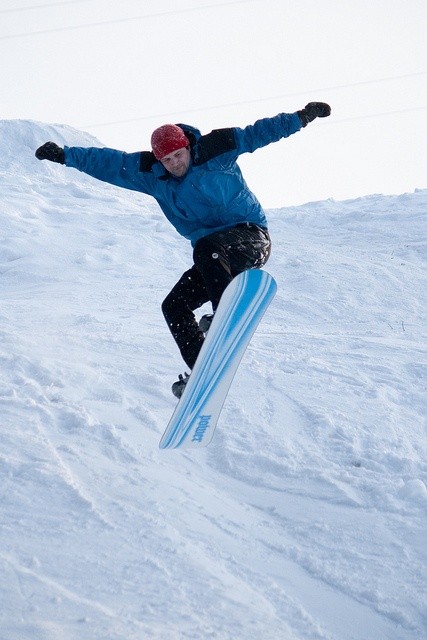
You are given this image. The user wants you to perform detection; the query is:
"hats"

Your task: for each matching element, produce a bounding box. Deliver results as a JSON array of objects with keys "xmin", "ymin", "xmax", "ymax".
[{"xmin": 150, "ymin": 124, "xmax": 187, "ymax": 159}]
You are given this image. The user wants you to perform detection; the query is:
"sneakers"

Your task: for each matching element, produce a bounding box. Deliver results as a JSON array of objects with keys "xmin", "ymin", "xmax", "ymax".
[
  {"xmin": 197, "ymin": 315, "xmax": 214, "ymax": 332},
  {"xmin": 172, "ymin": 376, "xmax": 189, "ymax": 397}
]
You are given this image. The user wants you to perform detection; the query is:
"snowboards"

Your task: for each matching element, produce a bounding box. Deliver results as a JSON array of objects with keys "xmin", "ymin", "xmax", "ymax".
[{"xmin": 160, "ymin": 269, "xmax": 277, "ymax": 450}]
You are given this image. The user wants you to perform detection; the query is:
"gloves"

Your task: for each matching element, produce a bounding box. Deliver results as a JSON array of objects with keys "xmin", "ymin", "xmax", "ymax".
[
  {"xmin": 297, "ymin": 102, "xmax": 331, "ymax": 128},
  {"xmin": 34, "ymin": 141, "xmax": 65, "ymax": 165}
]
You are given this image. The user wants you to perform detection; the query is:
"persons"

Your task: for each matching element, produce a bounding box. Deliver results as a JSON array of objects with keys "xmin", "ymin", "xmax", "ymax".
[{"xmin": 28, "ymin": 98, "xmax": 333, "ymax": 403}]
